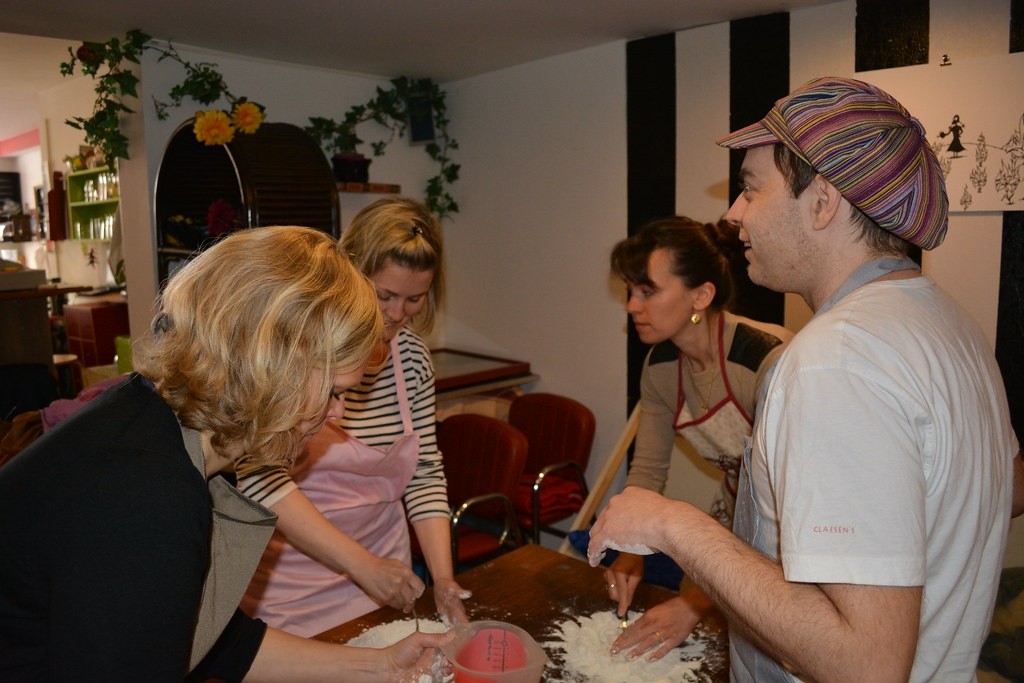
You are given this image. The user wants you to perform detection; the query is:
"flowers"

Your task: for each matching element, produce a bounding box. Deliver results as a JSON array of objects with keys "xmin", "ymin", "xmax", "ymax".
[
  {"xmin": 230, "ymin": 103, "xmax": 262, "ymax": 134},
  {"xmin": 193, "ymin": 110, "xmax": 236, "ymax": 145}
]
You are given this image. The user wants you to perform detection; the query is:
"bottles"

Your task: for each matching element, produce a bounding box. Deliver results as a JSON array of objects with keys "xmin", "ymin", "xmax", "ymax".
[{"xmin": 83, "ymin": 173, "xmax": 119, "ymax": 201}]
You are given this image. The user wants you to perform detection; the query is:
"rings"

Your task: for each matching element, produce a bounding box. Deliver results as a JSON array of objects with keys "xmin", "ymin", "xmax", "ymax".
[
  {"xmin": 653, "ymin": 631, "xmax": 662, "ymax": 641},
  {"xmin": 609, "ymin": 583, "xmax": 616, "ymax": 588}
]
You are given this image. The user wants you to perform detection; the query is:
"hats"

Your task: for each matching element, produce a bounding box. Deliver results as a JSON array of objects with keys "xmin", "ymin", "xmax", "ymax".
[{"xmin": 716, "ymin": 76, "xmax": 949, "ymax": 251}]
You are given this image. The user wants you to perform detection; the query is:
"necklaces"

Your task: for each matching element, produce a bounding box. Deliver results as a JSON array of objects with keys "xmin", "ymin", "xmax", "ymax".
[{"xmin": 684, "ymin": 312, "xmax": 716, "ymax": 417}]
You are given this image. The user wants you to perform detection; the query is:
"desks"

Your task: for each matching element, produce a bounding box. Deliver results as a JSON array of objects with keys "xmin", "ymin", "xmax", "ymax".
[
  {"xmin": 49, "ymin": 281, "xmax": 93, "ymax": 317},
  {"xmin": 307, "ymin": 542, "xmax": 730, "ymax": 683},
  {"xmin": 62, "ymin": 301, "xmax": 129, "ymax": 367},
  {"xmin": 430, "ymin": 346, "xmax": 540, "ymax": 402}
]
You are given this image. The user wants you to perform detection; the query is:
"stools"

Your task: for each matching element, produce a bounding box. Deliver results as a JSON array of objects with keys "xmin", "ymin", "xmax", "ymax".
[{"xmin": 53, "ymin": 353, "xmax": 88, "ymax": 396}]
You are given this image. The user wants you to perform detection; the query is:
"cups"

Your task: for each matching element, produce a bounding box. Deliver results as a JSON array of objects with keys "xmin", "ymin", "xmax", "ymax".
[{"xmin": 74, "ymin": 214, "xmax": 115, "ymax": 239}]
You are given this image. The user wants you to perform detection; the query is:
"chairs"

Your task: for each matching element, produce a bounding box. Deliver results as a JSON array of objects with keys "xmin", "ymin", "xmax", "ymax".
[
  {"xmin": 507, "ymin": 392, "xmax": 597, "ymax": 547},
  {"xmin": 426, "ymin": 413, "xmax": 530, "ymax": 586}
]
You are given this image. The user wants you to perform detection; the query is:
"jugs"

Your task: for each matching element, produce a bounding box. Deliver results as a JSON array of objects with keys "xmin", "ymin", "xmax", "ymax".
[{"xmin": 438, "ymin": 621, "xmax": 547, "ymax": 683}]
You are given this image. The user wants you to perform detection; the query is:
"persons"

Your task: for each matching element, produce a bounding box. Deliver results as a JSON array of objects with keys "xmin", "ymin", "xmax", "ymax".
[
  {"xmin": 0, "ymin": 221, "xmax": 463, "ymax": 683},
  {"xmin": 588, "ymin": 74, "xmax": 1024, "ymax": 683},
  {"xmin": 233, "ymin": 196, "xmax": 471, "ymax": 646},
  {"xmin": 598, "ymin": 210, "xmax": 796, "ymax": 662}
]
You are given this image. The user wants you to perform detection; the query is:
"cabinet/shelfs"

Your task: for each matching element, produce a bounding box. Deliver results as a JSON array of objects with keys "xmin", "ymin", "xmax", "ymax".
[{"xmin": 66, "ymin": 165, "xmax": 120, "ymax": 242}]
